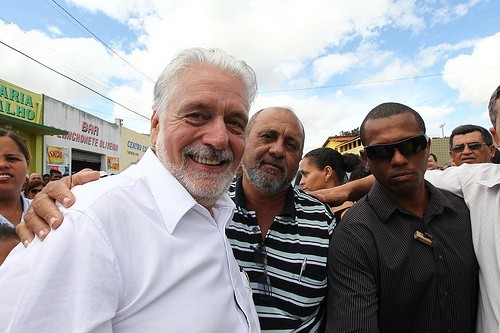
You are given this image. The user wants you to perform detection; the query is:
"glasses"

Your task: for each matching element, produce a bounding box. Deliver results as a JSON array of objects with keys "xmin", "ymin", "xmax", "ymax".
[
  {"xmin": 363, "ymin": 134, "xmax": 430, "ymax": 161},
  {"xmin": 449, "ymin": 141, "xmax": 490, "ymax": 154},
  {"xmin": 30, "ymin": 189, "xmax": 43, "ymax": 194}
]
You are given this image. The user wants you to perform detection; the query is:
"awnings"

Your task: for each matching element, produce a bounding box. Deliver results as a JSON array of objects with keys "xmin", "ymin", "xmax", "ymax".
[{"xmin": 0, "ymin": 113, "xmax": 68, "ymax": 136}]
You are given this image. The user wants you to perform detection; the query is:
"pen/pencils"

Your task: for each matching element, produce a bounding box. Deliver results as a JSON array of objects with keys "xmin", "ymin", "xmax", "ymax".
[
  {"xmin": 240, "ymin": 266, "xmax": 251, "ymax": 290},
  {"xmin": 300, "ymin": 258, "xmax": 306, "ymax": 282}
]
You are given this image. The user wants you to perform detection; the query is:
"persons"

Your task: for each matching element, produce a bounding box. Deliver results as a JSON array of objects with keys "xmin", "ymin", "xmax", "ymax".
[
  {"xmin": 0, "ymin": 46, "xmax": 260, "ymax": 333},
  {"xmin": 0, "ymin": 85, "xmax": 500, "ymax": 333}
]
